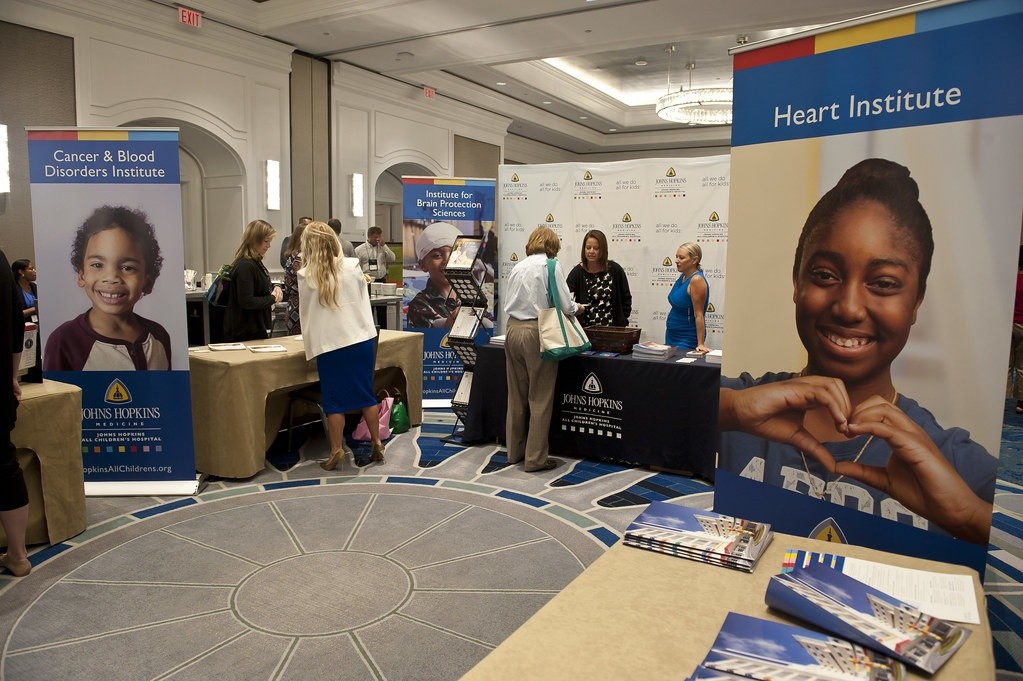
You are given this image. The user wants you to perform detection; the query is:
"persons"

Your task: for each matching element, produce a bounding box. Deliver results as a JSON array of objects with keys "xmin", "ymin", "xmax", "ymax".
[
  {"xmin": 503, "ymin": 225, "xmax": 585, "ymax": 475},
  {"xmin": 224, "ymin": 220, "xmax": 283, "ymax": 342},
  {"xmin": 12, "ymin": 260, "xmax": 38, "ymax": 317},
  {"xmin": 43, "ymin": 203, "xmax": 172, "ymax": 370},
  {"xmin": 354, "ymin": 226, "xmax": 396, "ymax": 329},
  {"xmin": 566, "ymin": 230, "xmax": 632, "ymax": 327},
  {"xmin": 327, "ymin": 219, "xmax": 357, "ymax": 257},
  {"xmin": 407, "ymin": 221, "xmax": 493, "ymax": 327},
  {"xmin": 297, "ymin": 220, "xmax": 385, "ymax": 471},
  {"xmin": 285, "ymin": 222, "xmax": 310, "ymax": 335},
  {"xmin": 280, "ymin": 216, "xmax": 312, "ymax": 268},
  {"xmin": 719, "ymin": 156, "xmax": 998, "ymax": 546},
  {"xmin": 0, "ymin": 248, "xmax": 33, "ymax": 577},
  {"xmin": 665, "ymin": 242, "xmax": 710, "ymax": 352}
]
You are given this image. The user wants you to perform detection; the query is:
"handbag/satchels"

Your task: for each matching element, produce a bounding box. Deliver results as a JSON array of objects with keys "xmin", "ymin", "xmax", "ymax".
[
  {"xmin": 203, "ymin": 258, "xmax": 243, "ymax": 307},
  {"xmin": 537, "ymin": 258, "xmax": 592, "ymax": 362},
  {"xmin": 376, "ymin": 387, "xmax": 409, "ymax": 434},
  {"xmin": 353, "ymin": 395, "xmax": 391, "ymax": 440}
]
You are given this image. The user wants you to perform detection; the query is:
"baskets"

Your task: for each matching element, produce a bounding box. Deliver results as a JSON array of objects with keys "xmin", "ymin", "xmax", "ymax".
[{"xmin": 583, "ymin": 326, "xmax": 642, "ymax": 355}]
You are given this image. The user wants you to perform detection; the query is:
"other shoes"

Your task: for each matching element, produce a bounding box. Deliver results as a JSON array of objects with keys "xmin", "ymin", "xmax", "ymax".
[
  {"xmin": 0, "ymin": 552, "xmax": 32, "ymax": 577},
  {"xmin": 528, "ymin": 460, "xmax": 557, "ymax": 472}
]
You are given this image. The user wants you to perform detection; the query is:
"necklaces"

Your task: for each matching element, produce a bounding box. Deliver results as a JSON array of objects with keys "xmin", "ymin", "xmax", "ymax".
[{"xmin": 798, "ymin": 371, "xmax": 898, "ymax": 495}]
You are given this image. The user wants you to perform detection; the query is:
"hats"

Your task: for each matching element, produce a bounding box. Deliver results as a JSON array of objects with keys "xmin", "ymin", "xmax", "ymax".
[{"xmin": 415, "ymin": 220, "xmax": 463, "ymax": 260}]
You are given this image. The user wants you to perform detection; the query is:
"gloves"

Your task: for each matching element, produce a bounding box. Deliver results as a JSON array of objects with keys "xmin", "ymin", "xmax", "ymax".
[{"xmin": 271, "ymin": 285, "xmax": 283, "ymax": 302}]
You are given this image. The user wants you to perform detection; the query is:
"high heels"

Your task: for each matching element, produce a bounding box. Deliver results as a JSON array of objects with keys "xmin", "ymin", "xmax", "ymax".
[
  {"xmin": 320, "ymin": 449, "xmax": 345, "ymax": 471},
  {"xmin": 371, "ymin": 443, "xmax": 385, "ymax": 463}
]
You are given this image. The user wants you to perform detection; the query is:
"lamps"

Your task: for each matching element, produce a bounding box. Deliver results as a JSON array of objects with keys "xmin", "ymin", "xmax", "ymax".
[
  {"xmin": 265, "ymin": 159, "xmax": 280, "ymax": 210},
  {"xmin": 350, "ymin": 173, "xmax": 363, "ymax": 218},
  {"xmin": 656, "ymin": 36, "xmax": 748, "ymax": 125}
]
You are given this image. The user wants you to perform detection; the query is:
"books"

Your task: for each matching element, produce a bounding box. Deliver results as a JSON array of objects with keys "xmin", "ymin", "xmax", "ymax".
[{"xmin": 623, "ymin": 500, "xmax": 973, "ymax": 681}]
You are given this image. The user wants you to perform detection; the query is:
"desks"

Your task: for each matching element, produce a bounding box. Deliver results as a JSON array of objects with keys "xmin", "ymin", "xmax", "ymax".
[
  {"xmin": 0, "ymin": 379, "xmax": 87, "ymax": 547},
  {"xmin": 457, "ymin": 531, "xmax": 996, "ymax": 681},
  {"xmin": 188, "ymin": 329, "xmax": 425, "ymax": 478},
  {"xmin": 370, "ymin": 295, "xmax": 403, "ymax": 331},
  {"xmin": 186, "ymin": 287, "xmax": 210, "ymax": 345},
  {"xmin": 461, "ymin": 345, "xmax": 722, "ymax": 483}
]
